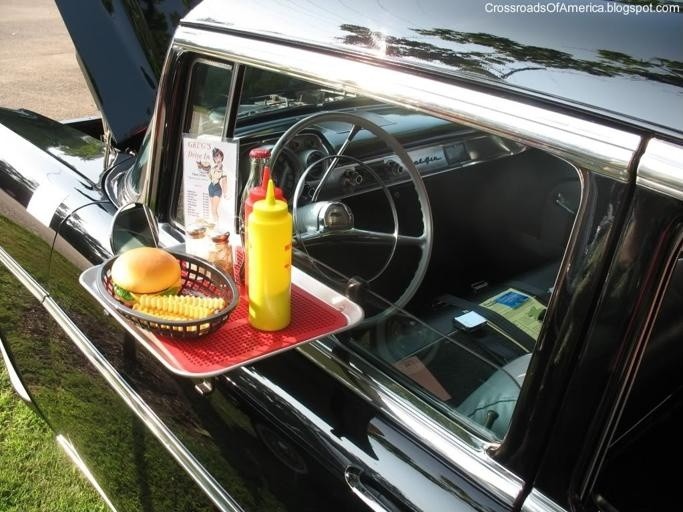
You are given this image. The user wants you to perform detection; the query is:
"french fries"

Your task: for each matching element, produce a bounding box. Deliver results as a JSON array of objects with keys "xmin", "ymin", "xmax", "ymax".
[{"xmin": 131, "ymin": 293, "xmax": 225, "ymax": 331}]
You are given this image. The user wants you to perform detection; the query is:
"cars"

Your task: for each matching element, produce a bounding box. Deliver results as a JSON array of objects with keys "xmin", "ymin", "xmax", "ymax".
[{"xmin": 0, "ymin": 0, "xmax": 682, "ymax": 512}]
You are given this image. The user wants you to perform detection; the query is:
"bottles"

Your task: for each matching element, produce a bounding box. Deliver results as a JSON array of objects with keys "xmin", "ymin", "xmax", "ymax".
[
  {"xmin": 208, "ymin": 233, "xmax": 232, "ymax": 270},
  {"xmin": 238, "ymin": 150, "xmax": 293, "ymax": 332}
]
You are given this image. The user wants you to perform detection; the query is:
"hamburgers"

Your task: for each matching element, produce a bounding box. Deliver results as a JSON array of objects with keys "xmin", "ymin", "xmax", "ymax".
[{"xmin": 111, "ymin": 247, "xmax": 182, "ymax": 305}]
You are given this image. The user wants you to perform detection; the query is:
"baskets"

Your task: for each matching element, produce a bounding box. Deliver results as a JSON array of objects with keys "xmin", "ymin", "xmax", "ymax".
[{"xmin": 98, "ymin": 247, "xmax": 241, "ymax": 340}]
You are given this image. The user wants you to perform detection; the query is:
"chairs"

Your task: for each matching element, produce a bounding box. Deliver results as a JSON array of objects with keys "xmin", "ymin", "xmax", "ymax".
[{"xmin": 395, "ymin": 256, "xmax": 552, "ymax": 441}]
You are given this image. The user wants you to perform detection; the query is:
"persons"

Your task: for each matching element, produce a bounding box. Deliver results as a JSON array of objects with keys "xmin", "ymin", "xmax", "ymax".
[{"xmin": 196, "ymin": 146, "xmax": 228, "ymax": 221}]
